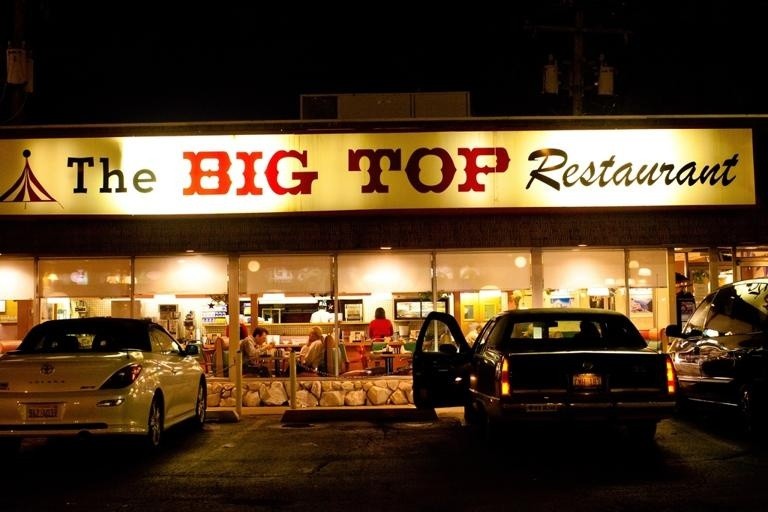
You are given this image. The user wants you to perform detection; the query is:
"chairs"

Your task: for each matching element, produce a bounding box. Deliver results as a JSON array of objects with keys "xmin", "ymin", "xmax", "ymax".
[
  {"xmin": 204, "ymin": 337, "xmax": 261, "ymax": 378},
  {"xmin": 296, "ymin": 335, "xmax": 372, "ymax": 378},
  {"xmin": 52, "ymin": 334, "xmax": 123, "ymax": 353},
  {"xmin": 564, "ymin": 322, "xmax": 603, "ymax": 346}
]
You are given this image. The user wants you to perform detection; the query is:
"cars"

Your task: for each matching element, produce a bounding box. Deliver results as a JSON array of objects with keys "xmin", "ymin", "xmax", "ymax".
[
  {"xmin": 411, "ymin": 308, "xmax": 681, "ymax": 450},
  {"xmin": 0, "ymin": 316, "xmax": 209, "ymax": 461}
]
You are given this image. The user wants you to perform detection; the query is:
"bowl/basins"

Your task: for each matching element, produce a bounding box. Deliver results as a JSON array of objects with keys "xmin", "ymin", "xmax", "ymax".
[{"xmin": 373, "ymin": 342, "xmax": 387, "ymax": 350}]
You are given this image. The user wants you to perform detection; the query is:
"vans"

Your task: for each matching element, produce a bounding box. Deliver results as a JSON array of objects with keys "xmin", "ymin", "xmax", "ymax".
[{"xmin": 666, "ymin": 278, "xmax": 768, "ymax": 445}]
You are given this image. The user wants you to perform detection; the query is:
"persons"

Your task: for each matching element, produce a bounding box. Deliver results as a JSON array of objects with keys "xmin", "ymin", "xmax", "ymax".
[
  {"xmin": 284, "ymin": 326, "xmax": 324, "ymax": 377},
  {"xmin": 310, "ymin": 301, "xmax": 334, "ymax": 323},
  {"xmin": 369, "ymin": 308, "xmax": 393, "ymax": 342},
  {"xmin": 240, "ymin": 327, "xmax": 270, "ymax": 377},
  {"xmin": 465, "ymin": 326, "xmax": 483, "ymax": 344}
]
